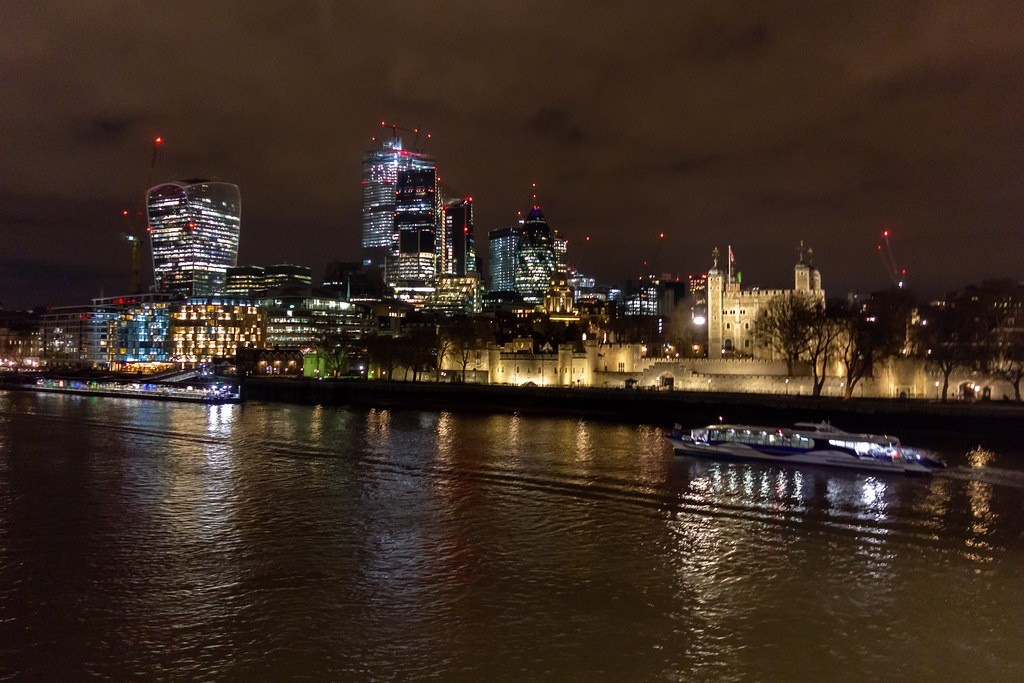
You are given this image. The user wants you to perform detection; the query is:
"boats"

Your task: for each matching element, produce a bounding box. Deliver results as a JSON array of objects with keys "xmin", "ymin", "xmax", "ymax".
[{"xmin": 664, "ymin": 415, "xmax": 948, "ymax": 475}]
